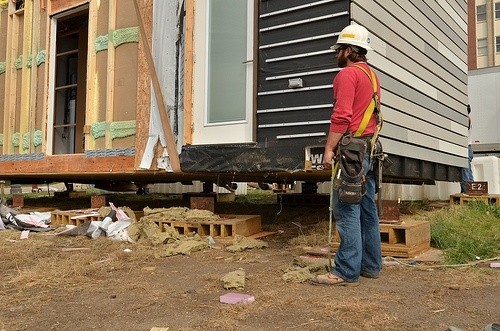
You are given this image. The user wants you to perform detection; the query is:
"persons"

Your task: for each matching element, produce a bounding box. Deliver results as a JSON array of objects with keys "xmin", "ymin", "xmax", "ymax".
[
  {"xmin": 311, "ymin": 24, "xmax": 382, "ymax": 287},
  {"xmin": 461, "ymin": 104, "xmax": 474, "ymax": 193}
]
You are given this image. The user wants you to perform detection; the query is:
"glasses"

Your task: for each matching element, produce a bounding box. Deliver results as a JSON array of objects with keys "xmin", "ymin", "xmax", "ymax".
[{"xmin": 335, "ymin": 48, "xmax": 345, "ymax": 53}]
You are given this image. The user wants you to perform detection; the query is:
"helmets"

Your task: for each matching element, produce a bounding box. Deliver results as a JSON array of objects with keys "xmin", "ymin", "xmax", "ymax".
[{"xmin": 329, "ymin": 24, "xmax": 372, "ymax": 50}]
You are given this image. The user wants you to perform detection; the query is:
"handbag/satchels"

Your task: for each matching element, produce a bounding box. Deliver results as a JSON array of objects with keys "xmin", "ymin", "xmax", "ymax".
[{"xmin": 339, "ymin": 133, "xmax": 366, "ymax": 178}]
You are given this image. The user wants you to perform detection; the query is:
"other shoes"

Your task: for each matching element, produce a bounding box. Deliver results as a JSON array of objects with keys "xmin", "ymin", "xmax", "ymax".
[
  {"xmin": 361, "ymin": 271, "xmax": 377, "ymax": 278},
  {"xmin": 309, "ymin": 273, "xmax": 358, "ymax": 286}
]
